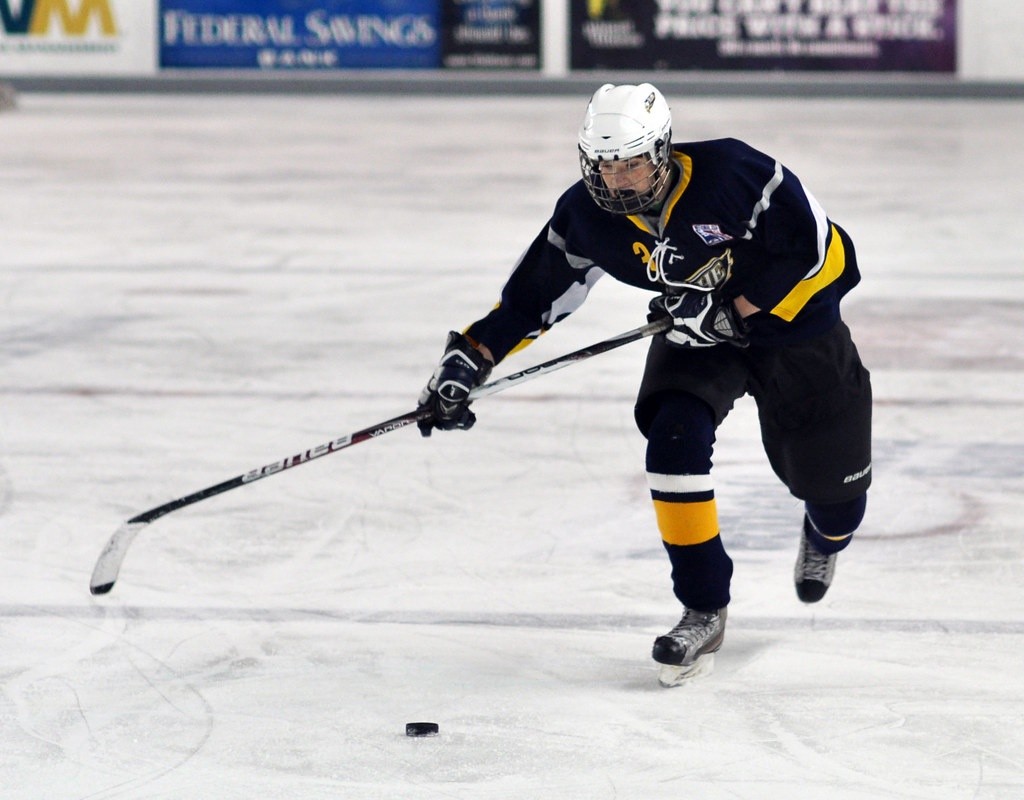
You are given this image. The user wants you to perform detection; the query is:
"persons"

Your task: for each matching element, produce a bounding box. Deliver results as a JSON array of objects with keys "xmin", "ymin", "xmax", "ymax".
[{"xmin": 417, "ymin": 82, "xmax": 874, "ymax": 689}]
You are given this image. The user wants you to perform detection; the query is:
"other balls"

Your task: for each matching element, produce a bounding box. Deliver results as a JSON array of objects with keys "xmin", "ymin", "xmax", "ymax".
[{"xmin": 407, "ymin": 723, "xmax": 440, "ymax": 739}]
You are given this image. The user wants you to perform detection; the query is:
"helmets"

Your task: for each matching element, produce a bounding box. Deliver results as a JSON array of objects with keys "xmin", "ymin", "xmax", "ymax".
[{"xmin": 577, "ymin": 83, "xmax": 672, "ymax": 216}]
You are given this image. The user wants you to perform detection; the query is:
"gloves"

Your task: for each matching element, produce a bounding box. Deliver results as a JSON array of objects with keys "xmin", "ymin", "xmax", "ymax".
[
  {"xmin": 647, "ymin": 291, "xmax": 750, "ymax": 348},
  {"xmin": 416, "ymin": 331, "xmax": 494, "ymax": 437}
]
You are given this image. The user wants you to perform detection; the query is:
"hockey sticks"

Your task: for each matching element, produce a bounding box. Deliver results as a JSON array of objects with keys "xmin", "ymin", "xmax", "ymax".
[{"xmin": 88, "ymin": 316, "xmax": 673, "ymax": 597}]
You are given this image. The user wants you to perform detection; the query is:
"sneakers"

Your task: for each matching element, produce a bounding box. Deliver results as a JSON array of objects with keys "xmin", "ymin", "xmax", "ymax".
[
  {"xmin": 793, "ymin": 526, "xmax": 837, "ymax": 604},
  {"xmin": 652, "ymin": 606, "xmax": 727, "ymax": 687}
]
